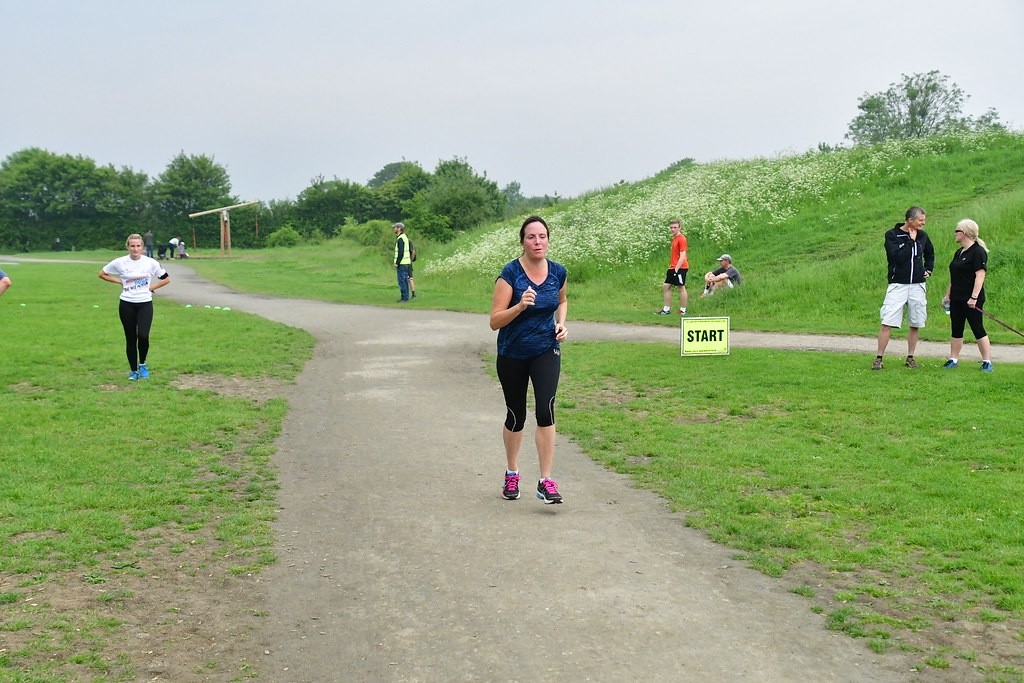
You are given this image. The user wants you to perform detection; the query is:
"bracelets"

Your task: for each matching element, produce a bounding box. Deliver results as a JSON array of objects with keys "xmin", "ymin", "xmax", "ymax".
[{"xmin": 970, "ymin": 296, "xmax": 978, "ymax": 300}]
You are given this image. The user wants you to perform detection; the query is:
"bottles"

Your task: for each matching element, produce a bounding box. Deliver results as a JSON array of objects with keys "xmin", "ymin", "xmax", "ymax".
[
  {"xmin": 706, "ymin": 279, "xmax": 711, "ymax": 289},
  {"xmin": 944, "ymin": 301, "xmax": 950, "ymax": 315}
]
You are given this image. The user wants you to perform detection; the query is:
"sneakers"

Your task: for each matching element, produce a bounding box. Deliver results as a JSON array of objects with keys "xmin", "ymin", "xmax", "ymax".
[
  {"xmin": 129, "ymin": 371, "xmax": 139, "ymax": 381},
  {"xmin": 139, "ymin": 363, "xmax": 150, "ymax": 379},
  {"xmin": 501, "ymin": 469, "xmax": 521, "ymax": 500},
  {"xmin": 537, "ymin": 477, "xmax": 563, "ymax": 504}
]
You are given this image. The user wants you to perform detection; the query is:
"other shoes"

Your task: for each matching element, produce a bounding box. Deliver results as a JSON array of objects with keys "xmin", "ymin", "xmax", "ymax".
[
  {"xmin": 942, "ymin": 357, "xmax": 959, "ymax": 368},
  {"xmin": 653, "ymin": 309, "xmax": 671, "ymax": 315},
  {"xmin": 700, "ymin": 290, "xmax": 713, "ymax": 297},
  {"xmin": 905, "ymin": 358, "xmax": 918, "ymax": 368},
  {"xmin": 978, "ymin": 361, "xmax": 992, "ymax": 370},
  {"xmin": 676, "ymin": 309, "xmax": 687, "ymax": 315},
  {"xmin": 872, "ymin": 359, "xmax": 883, "ymax": 370}
]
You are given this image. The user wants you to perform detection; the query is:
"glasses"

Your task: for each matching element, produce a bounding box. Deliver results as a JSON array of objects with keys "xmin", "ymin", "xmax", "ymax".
[{"xmin": 955, "ymin": 230, "xmax": 963, "ymax": 233}]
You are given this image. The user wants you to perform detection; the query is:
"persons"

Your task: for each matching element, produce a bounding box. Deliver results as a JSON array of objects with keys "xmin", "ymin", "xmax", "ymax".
[
  {"xmin": 143, "ymin": 229, "xmax": 185, "ymax": 260},
  {"xmin": 390, "ymin": 222, "xmax": 417, "ymax": 303},
  {"xmin": 700, "ymin": 254, "xmax": 742, "ymax": 300},
  {"xmin": 98, "ymin": 233, "xmax": 171, "ymax": 381},
  {"xmin": 942, "ymin": 218, "xmax": 994, "ymax": 373},
  {"xmin": 490, "ymin": 216, "xmax": 583, "ymax": 505},
  {"xmin": 654, "ymin": 219, "xmax": 690, "ymax": 315},
  {"xmin": 872, "ymin": 206, "xmax": 935, "ymax": 369},
  {"xmin": 0, "ymin": 270, "xmax": 12, "ymax": 298}
]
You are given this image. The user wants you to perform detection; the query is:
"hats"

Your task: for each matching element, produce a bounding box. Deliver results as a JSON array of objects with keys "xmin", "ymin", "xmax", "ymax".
[
  {"xmin": 392, "ymin": 223, "xmax": 405, "ymax": 231},
  {"xmin": 717, "ymin": 254, "xmax": 732, "ymax": 262}
]
